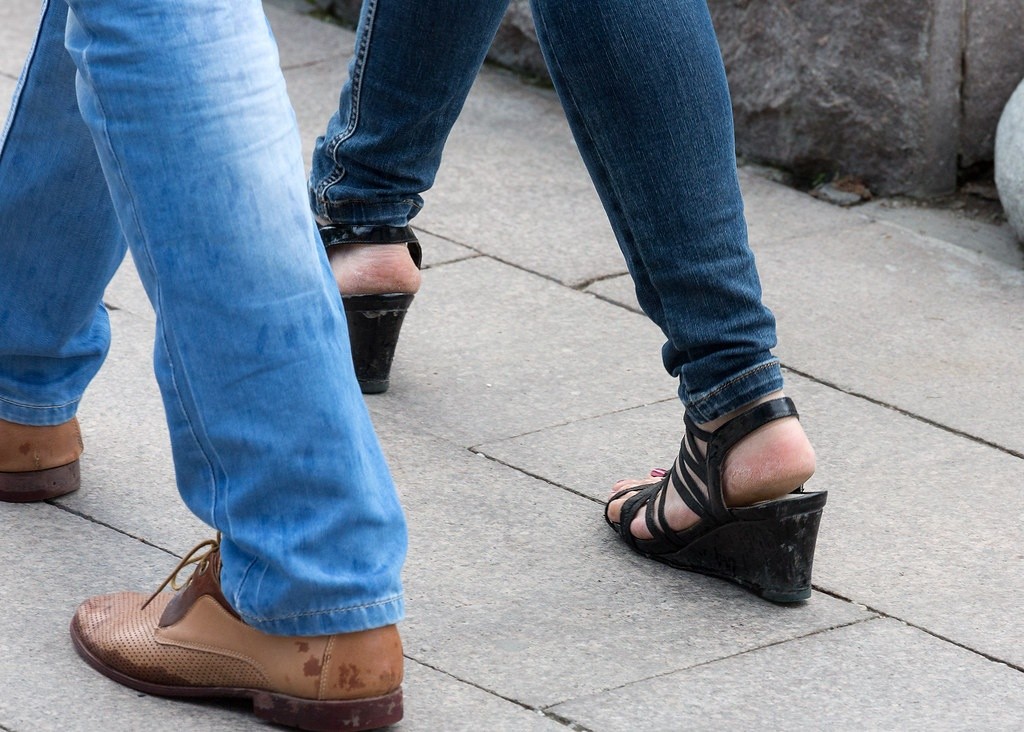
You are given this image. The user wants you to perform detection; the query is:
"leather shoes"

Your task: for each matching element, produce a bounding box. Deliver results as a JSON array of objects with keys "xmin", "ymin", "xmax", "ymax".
[
  {"xmin": 0, "ymin": 417, "xmax": 84, "ymax": 501},
  {"xmin": 70, "ymin": 530, "xmax": 404, "ymax": 731}
]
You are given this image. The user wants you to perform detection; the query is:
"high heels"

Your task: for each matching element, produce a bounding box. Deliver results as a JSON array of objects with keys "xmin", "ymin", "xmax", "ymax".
[
  {"xmin": 317, "ymin": 223, "xmax": 422, "ymax": 394},
  {"xmin": 604, "ymin": 396, "xmax": 828, "ymax": 602}
]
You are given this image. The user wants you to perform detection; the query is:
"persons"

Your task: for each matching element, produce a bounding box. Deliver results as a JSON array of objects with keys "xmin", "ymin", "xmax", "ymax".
[
  {"xmin": 0, "ymin": 0, "xmax": 408, "ymax": 731},
  {"xmin": 308, "ymin": 0, "xmax": 828, "ymax": 602}
]
